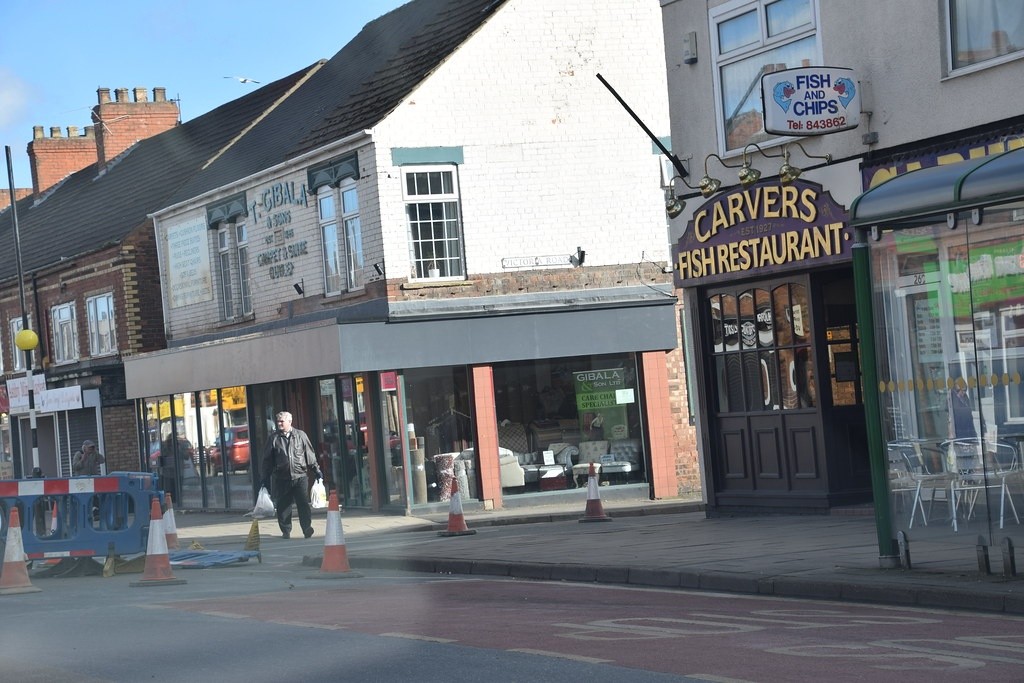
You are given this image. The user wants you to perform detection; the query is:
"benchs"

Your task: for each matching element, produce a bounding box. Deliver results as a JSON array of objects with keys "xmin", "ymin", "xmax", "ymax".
[{"xmin": 498, "ymin": 439, "xmax": 647, "ymax": 493}]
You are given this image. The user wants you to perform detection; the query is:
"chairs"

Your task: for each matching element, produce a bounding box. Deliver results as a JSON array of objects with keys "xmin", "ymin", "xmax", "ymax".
[{"xmin": 887, "ymin": 436, "xmax": 1024, "ymax": 532}]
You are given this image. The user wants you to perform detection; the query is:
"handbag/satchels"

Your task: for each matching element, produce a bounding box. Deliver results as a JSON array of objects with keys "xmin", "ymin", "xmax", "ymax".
[
  {"xmin": 310, "ymin": 478, "xmax": 327, "ymax": 509},
  {"xmin": 252, "ymin": 487, "xmax": 276, "ymax": 520}
]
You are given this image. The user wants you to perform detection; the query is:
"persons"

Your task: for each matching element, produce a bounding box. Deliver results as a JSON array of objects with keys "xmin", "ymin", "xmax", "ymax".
[
  {"xmin": 73, "ymin": 440, "xmax": 105, "ymax": 476},
  {"xmin": 261, "ymin": 411, "xmax": 323, "ymax": 538},
  {"xmin": 159, "ymin": 430, "xmax": 189, "ymax": 504},
  {"xmin": 947, "ymin": 379, "xmax": 978, "ymax": 485}
]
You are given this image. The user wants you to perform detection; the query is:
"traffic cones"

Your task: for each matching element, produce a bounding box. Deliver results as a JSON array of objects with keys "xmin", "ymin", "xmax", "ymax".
[
  {"xmin": 129, "ymin": 497, "xmax": 187, "ymax": 588},
  {"xmin": 305, "ymin": 489, "xmax": 364, "ymax": 579},
  {"xmin": 0, "ymin": 506, "xmax": 44, "ymax": 596},
  {"xmin": 161, "ymin": 492, "xmax": 182, "ymax": 552},
  {"xmin": 436, "ymin": 476, "xmax": 477, "ymax": 537},
  {"xmin": 578, "ymin": 462, "xmax": 613, "ymax": 522}
]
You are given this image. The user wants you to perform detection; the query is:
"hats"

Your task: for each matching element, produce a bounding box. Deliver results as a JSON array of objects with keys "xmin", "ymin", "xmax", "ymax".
[{"xmin": 83, "ymin": 440, "xmax": 95, "ymax": 448}]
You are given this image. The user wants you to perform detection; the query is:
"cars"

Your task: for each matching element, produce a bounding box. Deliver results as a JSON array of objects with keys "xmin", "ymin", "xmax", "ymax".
[{"xmin": 207, "ymin": 423, "xmax": 251, "ymax": 478}]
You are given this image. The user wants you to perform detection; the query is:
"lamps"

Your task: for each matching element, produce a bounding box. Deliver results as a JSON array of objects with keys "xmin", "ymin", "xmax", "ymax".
[
  {"xmin": 293, "ymin": 278, "xmax": 304, "ymax": 298},
  {"xmin": 775, "ymin": 142, "xmax": 832, "ymax": 187},
  {"xmin": 573, "ymin": 246, "xmax": 585, "ymax": 265},
  {"xmin": 698, "ymin": 154, "xmax": 755, "ymax": 198},
  {"xmin": 738, "ymin": 143, "xmax": 789, "ymax": 191},
  {"xmin": 666, "ymin": 176, "xmax": 701, "ymax": 219},
  {"xmin": 374, "ymin": 258, "xmax": 386, "ymax": 277}
]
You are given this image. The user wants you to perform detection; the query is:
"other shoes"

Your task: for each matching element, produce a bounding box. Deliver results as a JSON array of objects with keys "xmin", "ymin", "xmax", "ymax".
[
  {"xmin": 303, "ymin": 527, "xmax": 314, "ymax": 537},
  {"xmin": 94, "ymin": 515, "xmax": 99, "ymax": 522},
  {"xmin": 282, "ymin": 531, "xmax": 291, "ymax": 539}
]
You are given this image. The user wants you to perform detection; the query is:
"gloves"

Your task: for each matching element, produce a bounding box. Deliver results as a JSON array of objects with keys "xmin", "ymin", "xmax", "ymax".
[
  {"xmin": 261, "ymin": 481, "xmax": 268, "ymax": 487},
  {"xmin": 316, "ymin": 471, "xmax": 324, "ymax": 480}
]
